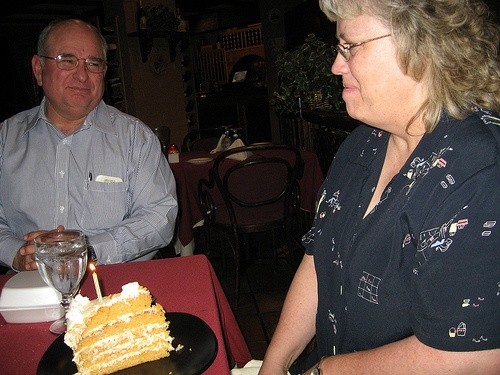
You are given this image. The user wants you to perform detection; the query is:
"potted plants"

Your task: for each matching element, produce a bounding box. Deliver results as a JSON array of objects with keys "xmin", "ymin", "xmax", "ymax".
[{"xmin": 270, "ymin": 34, "xmax": 346, "ymax": 114}]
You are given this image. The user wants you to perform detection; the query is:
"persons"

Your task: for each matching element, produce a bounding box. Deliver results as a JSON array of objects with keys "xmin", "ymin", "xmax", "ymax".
[
  {"xmin": 258, "ymin": 0, "xmax": 500, "ymax": 375},
  {"xmin": 0, "ymin": 20, "xmax": 179, "ymax": 272}
]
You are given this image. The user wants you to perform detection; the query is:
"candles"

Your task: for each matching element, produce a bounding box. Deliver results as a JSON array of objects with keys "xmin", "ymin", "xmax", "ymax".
[{"xmin": 89, "ymin": 263, "xmax": 103, "ymax": 303}]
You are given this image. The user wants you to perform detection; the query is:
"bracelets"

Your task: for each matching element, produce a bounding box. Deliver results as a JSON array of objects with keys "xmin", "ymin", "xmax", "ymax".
[
  {"xmin": 82, "ymin": 235, "xmax": 97, "ymax": 261},
  {"xmin": 310, "ymin": 356, "xmax": 326, "ymax": 375}
]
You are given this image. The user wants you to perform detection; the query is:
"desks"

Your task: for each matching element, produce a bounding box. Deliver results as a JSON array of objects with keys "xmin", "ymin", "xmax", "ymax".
[
  {"xmin": 0, "ymin": 254, "xmax": 253, "ymax": 375},
  {"xmin": 166, "ymin": 144, "xmax": 325, "ymax": 257}
]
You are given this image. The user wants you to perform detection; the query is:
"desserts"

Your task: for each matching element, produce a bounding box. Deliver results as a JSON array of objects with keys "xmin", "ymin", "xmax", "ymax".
[{"xmin": 64, "ymin": 282, "xmax": 176, "ymax": 375}]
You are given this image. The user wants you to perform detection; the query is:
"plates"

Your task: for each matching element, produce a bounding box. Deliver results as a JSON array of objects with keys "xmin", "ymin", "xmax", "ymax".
[
  {"xmin": 250, "ymin": 142, "xmax": 272, "ymax": 146},
  {"xmin": 187, "ymin": 158, "xmax": 212, "ymax": 164},
  {"xmin": 35, "ymin": 312, "xmax": 218, "ymax": 375}
]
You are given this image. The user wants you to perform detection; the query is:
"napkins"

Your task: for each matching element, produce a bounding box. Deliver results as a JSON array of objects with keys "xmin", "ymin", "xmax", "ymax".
[
  {"xmin": 211, "ymin": 133, "xmax": 239, "ymax": 154},
  {"xmin": 225, "ymin": 138, "xmax": 253, "ymax": 162}
]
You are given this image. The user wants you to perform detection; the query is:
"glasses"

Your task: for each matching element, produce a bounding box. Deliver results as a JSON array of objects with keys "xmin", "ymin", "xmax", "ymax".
[
  {"xmin": 330, "ymin": 34, "xmax": 391, "ymax": 62},
  {"xmin": 40, "ymin": 54, "xmax": 107, "ymax": 73}
]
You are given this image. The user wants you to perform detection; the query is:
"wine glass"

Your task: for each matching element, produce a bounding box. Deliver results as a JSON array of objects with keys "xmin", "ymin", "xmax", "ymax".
[
  {"xmin": 34, "ymin": 230, "xmax": 88, "ymax": 335},
  {"xmin": 220, "ymin": 125, "xmax": 242, "ymax": 152}
]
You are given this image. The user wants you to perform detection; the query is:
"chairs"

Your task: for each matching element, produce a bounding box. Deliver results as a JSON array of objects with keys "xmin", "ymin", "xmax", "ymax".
[
  {"xmin": 198, "ymin": 143, "xmax": 315, "ymax": 307},
  {"xmin": 181, "ymin": 129, "xmax": 221, "ymax": 153}
]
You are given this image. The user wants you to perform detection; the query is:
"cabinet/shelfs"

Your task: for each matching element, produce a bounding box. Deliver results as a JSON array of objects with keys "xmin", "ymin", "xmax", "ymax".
[{"xmin": 88, "ymin": 15, "xmax": 129, "ymax": 115}]
